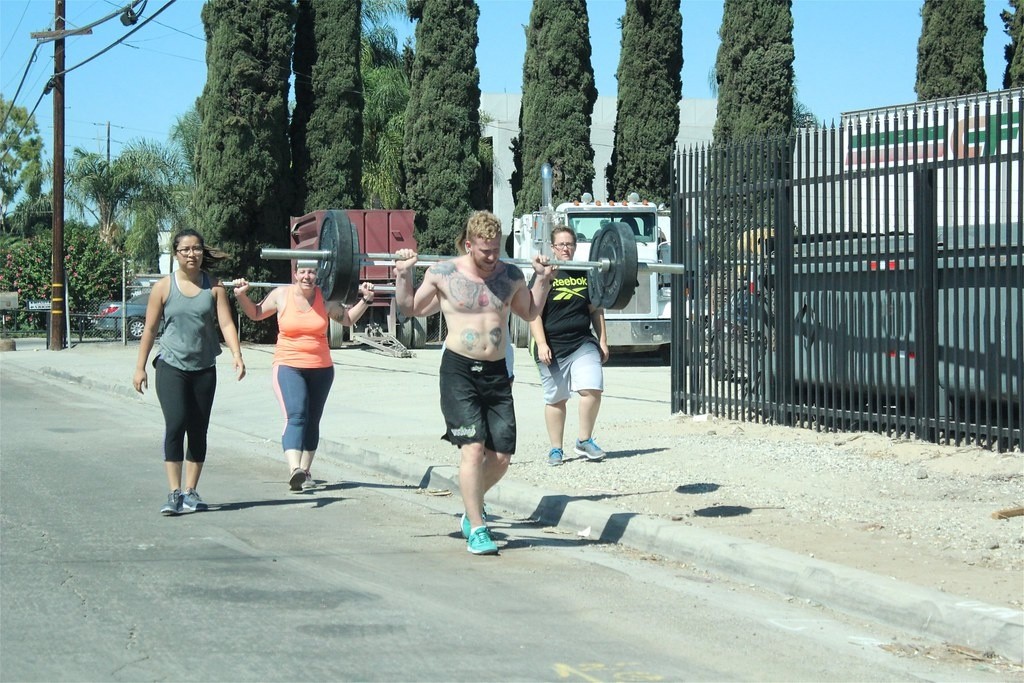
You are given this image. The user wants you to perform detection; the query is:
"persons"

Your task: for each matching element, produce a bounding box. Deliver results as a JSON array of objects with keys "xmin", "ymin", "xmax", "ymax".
[
  {"xmin": 527, "ymin": 225, "xmax": 609, "ymax": 466},
  {"xmin": 233, "ymin": 263, "xmax": 375, "ymax": 492},
  {"xmin": 396, "ymin": 210, "xmax": 552, "ymax": 555},
  {"xmin": 133, "ymin": 229, "xmax": 245, "ymax": 515}
]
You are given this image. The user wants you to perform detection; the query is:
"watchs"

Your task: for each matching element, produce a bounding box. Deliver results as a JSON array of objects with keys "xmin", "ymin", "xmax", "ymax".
[{"xmin": 362, "ymin": 297, "xmax": 374, "ymax": 304}]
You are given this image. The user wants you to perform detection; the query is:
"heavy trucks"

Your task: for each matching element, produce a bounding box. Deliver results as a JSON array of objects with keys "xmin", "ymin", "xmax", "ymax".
[{"xmin": 512, "ymin": 163, "xmax": 708, "ymax": 357}]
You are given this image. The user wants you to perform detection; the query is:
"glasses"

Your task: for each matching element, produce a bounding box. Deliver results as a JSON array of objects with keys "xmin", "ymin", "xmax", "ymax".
[
  {"xmin": 176, "ymin": 247, "xmax": 203, "ymax": 255},
  {"xmin": 554, "ymin": 242, "xmax": 576, "ymax": 249}
]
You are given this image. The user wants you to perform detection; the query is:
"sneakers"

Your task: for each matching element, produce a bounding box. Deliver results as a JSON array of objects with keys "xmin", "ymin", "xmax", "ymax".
[
  {"xmin": 575, "ymin": 438, "xmax": 606, "ymax": 459},
  {"xmin": 547, "ymin": 448, "xmax": 563, "ymax": 465},
  {"xmin": 460, "ymin": 512, "xmax": 471, "ymax": 538},
  {"xmin": 160, "ymin": 490, "xmax": 185, "ymax": 514},
  {"xmin": 467, "ymin": 528, "xmax": 499, "ymax": 555},
  {"xmin": 182, "ymin": 488, "xmax": 207, "ymax": 511}
]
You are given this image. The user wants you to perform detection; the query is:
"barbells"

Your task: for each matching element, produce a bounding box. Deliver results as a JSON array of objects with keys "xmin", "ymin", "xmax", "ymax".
[
  {"xmin": 257, "ymin": 208, "xmax": 687, "ymax": 310},
  {"xmin": 219, "ymin": 277, "xmax": 420, "ymax": 303}
]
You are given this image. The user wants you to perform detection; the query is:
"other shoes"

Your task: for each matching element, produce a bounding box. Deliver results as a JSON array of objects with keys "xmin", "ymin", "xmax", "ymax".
[
  {"xmin": 302, "ymin": 471, "xmax": 316, "ymax": 487},
  {"xmin": 289, "ymin": 467, "xmax": 306, "ymax": 491}
]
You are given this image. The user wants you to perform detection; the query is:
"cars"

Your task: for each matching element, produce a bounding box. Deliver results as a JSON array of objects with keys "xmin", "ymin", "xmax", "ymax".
[{"xmin": 95, "ymin": 293, "xmax": 151, "ymax": 340}]
